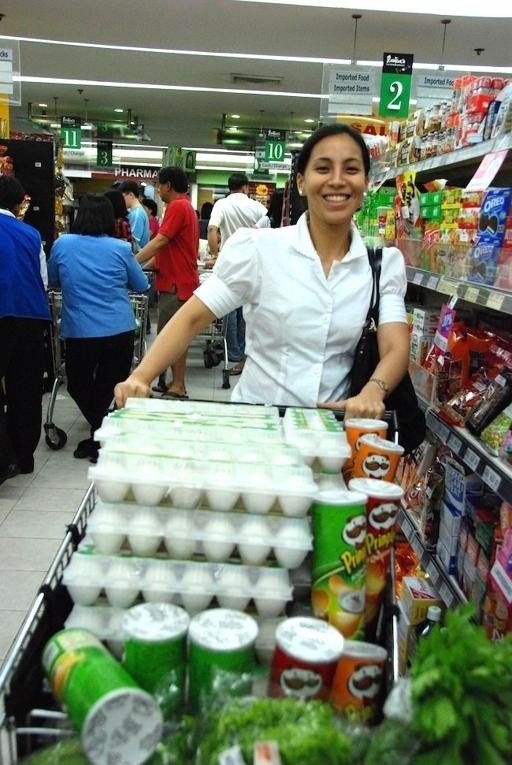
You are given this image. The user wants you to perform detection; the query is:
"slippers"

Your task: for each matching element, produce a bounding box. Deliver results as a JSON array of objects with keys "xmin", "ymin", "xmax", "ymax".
[
  {"xmin": 229, "ymin": 361, "xmax": 245, "ymax": 375},
  {"xmin": 152, "ymin": 383, "xmax": 189, "ymax": 399}
]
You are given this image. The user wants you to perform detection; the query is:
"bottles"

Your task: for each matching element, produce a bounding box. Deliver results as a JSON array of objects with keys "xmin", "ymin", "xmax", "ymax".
[{"xmin": 405, "ymin": 604, "xmax": 441, "ymax": 671}]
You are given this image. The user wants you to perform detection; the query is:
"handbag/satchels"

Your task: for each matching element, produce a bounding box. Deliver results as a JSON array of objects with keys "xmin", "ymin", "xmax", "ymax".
[{"xmin": 350, "ymin": 326, "xmax": 427, "ymax": 456}]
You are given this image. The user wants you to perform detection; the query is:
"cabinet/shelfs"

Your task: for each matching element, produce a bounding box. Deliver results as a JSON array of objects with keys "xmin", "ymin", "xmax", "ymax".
[
  {"xmin": 368, "ymin": 133, "xmax": 510, "ymax": 641},
  {"xmin": 0, "ymin": 140, "xmax": 56, "ymax": 394}
]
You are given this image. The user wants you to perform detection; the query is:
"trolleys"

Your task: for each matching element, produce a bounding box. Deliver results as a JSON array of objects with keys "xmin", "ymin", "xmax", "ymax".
[
  {"xmin": 41, "ymin": 288, "xmax": 155, "ymax": 449},
  {"xmin": 141, "ymin": 265, "xmax": 238, "ymax": 392},
  {"xmin": 1, "ymin": 388, "xmax": 411, "ymax": 765}
]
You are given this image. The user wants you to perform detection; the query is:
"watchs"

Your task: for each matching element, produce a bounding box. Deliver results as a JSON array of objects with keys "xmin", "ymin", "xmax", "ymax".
[{"xmin": 369, "ymin": 377, "xmax": 391, "ymax": 397}]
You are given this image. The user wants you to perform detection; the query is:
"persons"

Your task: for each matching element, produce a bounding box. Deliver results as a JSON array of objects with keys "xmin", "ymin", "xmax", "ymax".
[
  {"xmin": 0, "ymin": 176, "xmax": 53, "ymax": 481},
  {"xmin": 113, "ymin": 122, "xmax": 411, "ymax": 431},
  {"xmin": 133, "ymin": 166, "xmax": 202, "ymax": 401},
  {"xmin": 46, "ymin": 194, "xmax": 148, "ymax": 464},
  {"xmin": 105, "ymin": 180, "xmax": 159, "ymax": 254},
  {"xmin": 194, "ymin": 199, "xmax": 218, "ymax": 240},
  {"xmin": 206, "ymin": 173, "xmax": 285, "ymax": 362}
]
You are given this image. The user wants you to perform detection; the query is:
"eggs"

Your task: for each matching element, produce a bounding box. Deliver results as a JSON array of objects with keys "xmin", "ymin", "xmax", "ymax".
[{"xmin": 64, "ymin": 397, "xmax": 349, "ymax": 689}]
